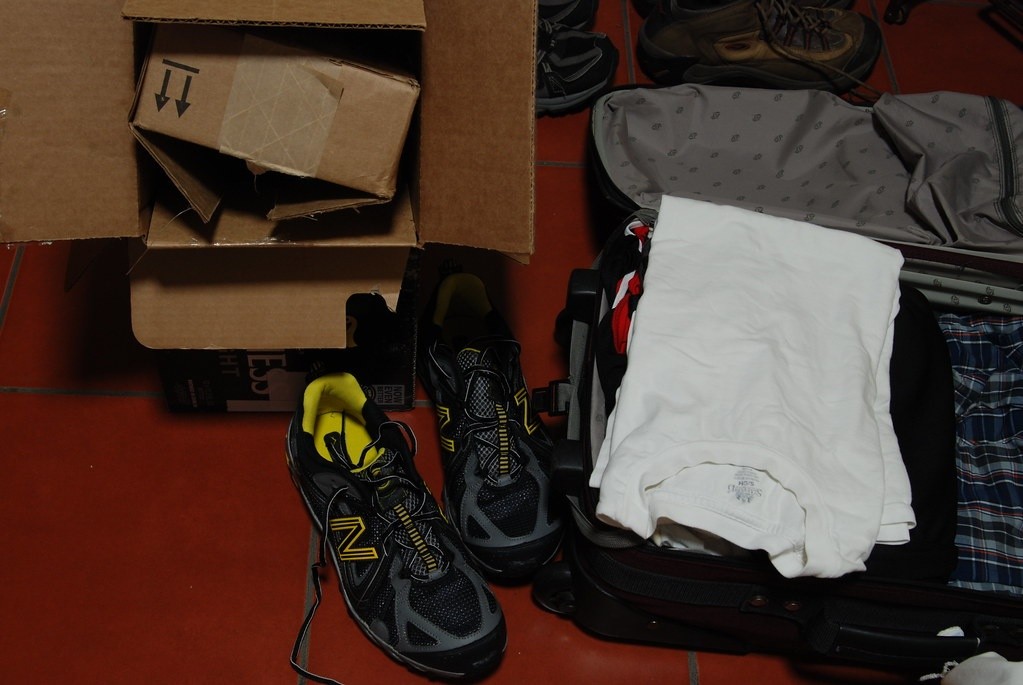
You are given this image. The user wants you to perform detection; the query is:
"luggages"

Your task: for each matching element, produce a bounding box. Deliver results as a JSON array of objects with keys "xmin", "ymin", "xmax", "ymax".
[{"xmin": 533, "ymin": 74, "xmax": 1023, "ymax": 673}]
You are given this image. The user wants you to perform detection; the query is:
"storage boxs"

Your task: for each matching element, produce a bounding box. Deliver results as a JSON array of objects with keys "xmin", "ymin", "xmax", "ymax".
[{"xmin": 0, "ymin": 0, "xmax": 536, "ymax": 415}]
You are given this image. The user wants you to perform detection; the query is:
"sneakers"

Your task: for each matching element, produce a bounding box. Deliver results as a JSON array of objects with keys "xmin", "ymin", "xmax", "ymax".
[
  {"xmin": 536, "ymin": 0, "xmax": 616, "ymax": 117},
  {"xmin": 285, "ymin": 372, "xmax": 508, "ymax": 685},
  {"xmin": 631, "ymin": 2, "xmax": 883, "ymax": 94},
  {"xmin": 420, "ymin": 269, "xmax": 566, "ymax": 580}
]
